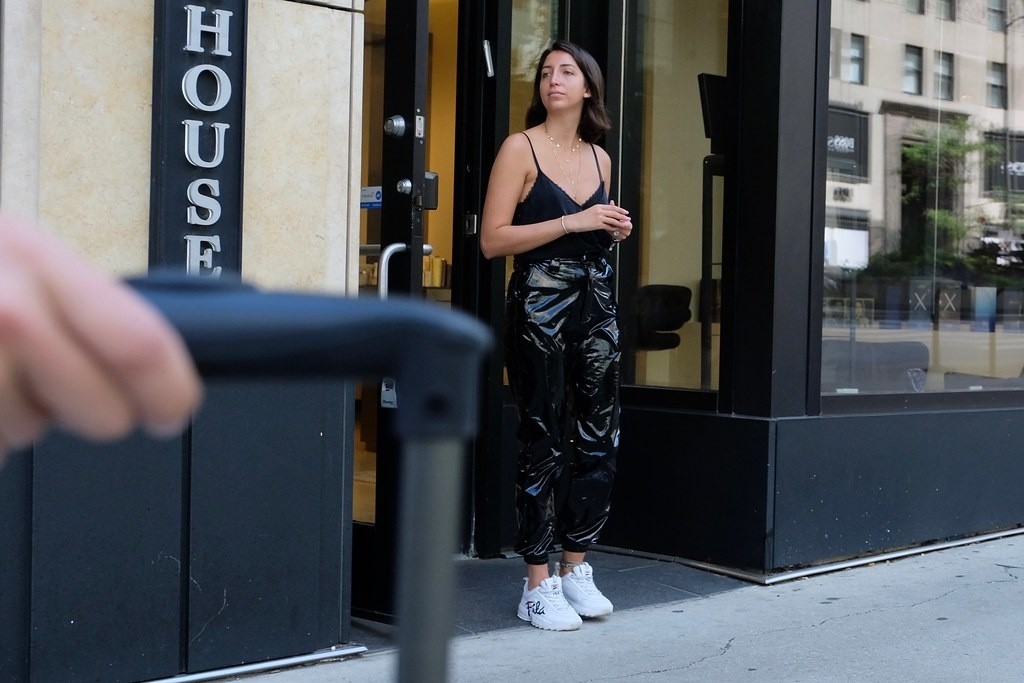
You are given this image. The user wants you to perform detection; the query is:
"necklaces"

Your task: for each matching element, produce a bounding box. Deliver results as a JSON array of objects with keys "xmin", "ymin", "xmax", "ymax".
[{"xmin": 543, "ymin": 123, "xmax": 582, "ymax": 199}]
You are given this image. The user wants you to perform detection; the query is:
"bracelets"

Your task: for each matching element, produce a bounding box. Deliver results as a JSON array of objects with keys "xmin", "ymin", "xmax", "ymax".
[{"xmin": 561, "ymin": 215, "xmax": 568, "ymax": 235}]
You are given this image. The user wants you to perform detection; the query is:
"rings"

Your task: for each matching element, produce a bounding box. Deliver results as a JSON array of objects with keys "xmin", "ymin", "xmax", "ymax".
[{"xmin": 612, "ymin": 230, "xmax": 620, "ymax": 237}]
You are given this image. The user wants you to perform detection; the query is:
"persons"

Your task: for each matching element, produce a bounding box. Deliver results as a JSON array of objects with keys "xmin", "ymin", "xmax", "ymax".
[
  {"xmin": 1, "ymin": 208, "xmax": 205, "ymax": 466},
  {"xmin": 481, "ymin": 40, "xmax": 633, "ymax": 632}
]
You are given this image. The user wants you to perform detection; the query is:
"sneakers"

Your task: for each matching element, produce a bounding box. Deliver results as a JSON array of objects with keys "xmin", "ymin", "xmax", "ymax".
[
  {"xmin": 517, "ymin": 575, "xmax": 582, "ymax": 631},
  {"xmin": 553, "ymin": 562, "xmax": 613, "ymax": 617}
]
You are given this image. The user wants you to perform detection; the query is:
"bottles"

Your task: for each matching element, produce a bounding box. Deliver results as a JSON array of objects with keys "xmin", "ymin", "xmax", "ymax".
[
  {"xmin": 441, "ymin": 258, "xmax": 451, "ymax": 288},
  {"xmin": 432, "ymin": 256, "xmax": 442, "ymax": 287}
]
